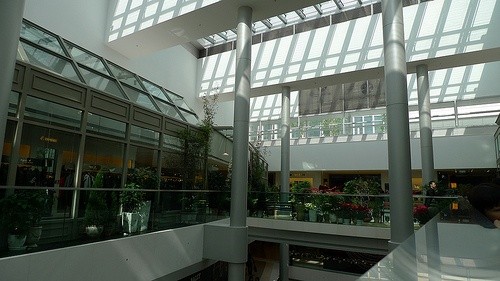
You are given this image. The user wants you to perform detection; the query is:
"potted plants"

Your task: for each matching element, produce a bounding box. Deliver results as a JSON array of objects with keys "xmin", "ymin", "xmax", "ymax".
[
  {"xmin": 83, "ymin": 168, "xmax": 110, "ymax": 238},
  {"xmin": 0, "ymin": 191, "xmax": 52, "ymax": 251},
  {"xmin": 296, "ymin": 203, "xmax": 344, "ymax": 224},
  {"xmin": 117, "ymin": 183, "xmax": 152, "ymax": 234}
]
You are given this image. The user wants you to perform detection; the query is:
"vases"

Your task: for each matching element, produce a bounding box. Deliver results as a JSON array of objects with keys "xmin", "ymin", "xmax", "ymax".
[
  {"xmin": 365, "ymin": 218, "xmax": 372, "ymax": 221},
  {"xmin": 354, "ymin": 219, "xmax": 362, "ymax": 226},
  {"xmin": 343, "ymin": 218, "xmax": 350, "ymax": 224}
]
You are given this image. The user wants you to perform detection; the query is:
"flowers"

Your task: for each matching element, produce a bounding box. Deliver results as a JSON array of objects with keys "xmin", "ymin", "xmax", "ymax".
[
  {"xmin": 342, "ymin": 203, "xmax": 368, "ymax": 220},
  {"xmin": 412, "ymin": 205, "xmax": 429, "ymax": 222}
]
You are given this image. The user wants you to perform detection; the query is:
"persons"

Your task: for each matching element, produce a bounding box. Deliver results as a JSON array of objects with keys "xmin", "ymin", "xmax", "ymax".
[
  {"xmin": 319, "ymin": 178, "xmax": 328, "ymax": 194},
  {"xmin": 469, "ymin": 183, "xmax": 500, "ymax": 229},
  {"xmin": 425, "ymin": 181, "xmax": 440, "ymax": 207}
]
